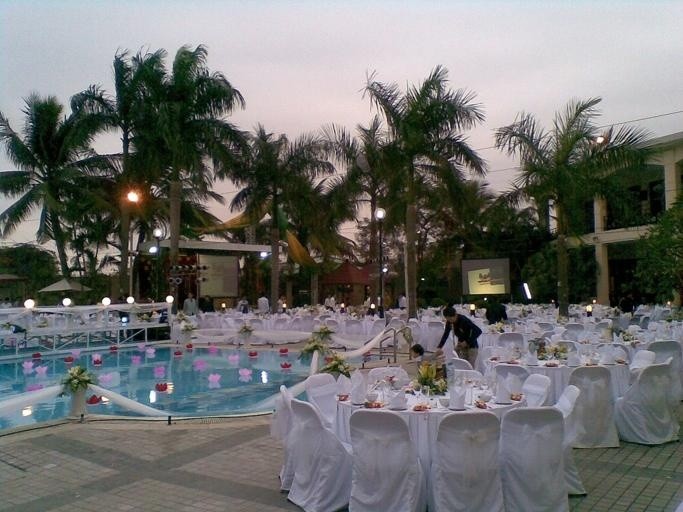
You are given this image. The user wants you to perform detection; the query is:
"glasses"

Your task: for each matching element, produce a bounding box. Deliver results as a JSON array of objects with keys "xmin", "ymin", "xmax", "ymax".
[{"xmin": 167, "ymin": 263, "xmax": 211, "ymax": 285}]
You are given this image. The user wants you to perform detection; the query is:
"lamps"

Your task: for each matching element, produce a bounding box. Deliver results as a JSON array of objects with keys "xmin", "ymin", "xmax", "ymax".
[
  {"xmin": 551, "ymin": 300, "xmax": 555, "ymax": 305},
  {"xmin": 340, "ymin": 303, "xmax": 344, "ymax": 313},
  {"xmin": 167, "ymin": 263, "xmax": 211, "ymax": 285},
  {"xmin": 22, "ymin": 406, "xmax": 32, "ymax": 417},
  {"xmin": 101, "ymin": 396, "xmax": 109, "ymax": 405},
  {"xmin": 150, "ymin": 390, "xmax": 157, "ymax": 404},
  {"xmin": 484, "ymin": 297, "xmax": 488, "ymax": 301},
  {"xmin": 261, "ymin": 370, "xmax": 268, "ymax": 384},
  {"xmin": 166, "ymin": 296, "xmax": 175, "ymax": 303},
  {"xmin": 166, "ymin": 382, "xmax": 174, "ymax": 394},
  {"xmin": 667, "ymin": 301, "xmax": 671, "ymax": 306},
  {"xmin": 24, "ymin": 299, "xmax": 35, "ymax": 308},
  {"xmin": 127, "ymin": 296, "xmax": 134, "ymax": 304},
  {"xmin": 222, "ymin": 303, "xmax": 226, "ymax": 312},
  {"xmin": 282, "ymin": 303, "xmax": 287, "ymax": 313},
  {"xmin": 63, "ymin": 298, "xmax": 72, "ymax": 307},
  {"xmin": 593, "ymin": 300, "xmax": 597, "ymax": 304},
  {"xmin": 102, "ymin": 297, "xmax": 111, "ymax": 306},
  {"xmin": 371, "ymin": 303, "xmax": 375, "ymax": 315}
]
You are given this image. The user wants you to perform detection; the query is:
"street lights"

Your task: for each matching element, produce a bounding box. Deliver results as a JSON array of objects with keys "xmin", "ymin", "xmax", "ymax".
[{"xmin": 454, "ymin": 345, "xmax": 470, "ymax": 361}]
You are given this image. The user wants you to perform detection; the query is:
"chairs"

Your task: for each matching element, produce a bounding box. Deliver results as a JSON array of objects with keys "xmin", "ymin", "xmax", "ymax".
[
  {"xmin": 278, "ymin": 368, "xmax": 586, "ymax": 511},
  {"xmin": 1, "ymin": 308, "xmax": 412, "ymax": 346},
  {"xmin": 410, "ymin": 303, "xmax": 681, "ymax": 448}
]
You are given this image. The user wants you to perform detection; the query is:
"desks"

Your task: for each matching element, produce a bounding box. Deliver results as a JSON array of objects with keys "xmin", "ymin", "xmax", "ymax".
[
  {"xmin": 152, "ymin": 227, "xmax": 163, "ymax": 302},
  {"xmin": 372, "ymin": 205, "xmax": 386, "ymax": 319}
]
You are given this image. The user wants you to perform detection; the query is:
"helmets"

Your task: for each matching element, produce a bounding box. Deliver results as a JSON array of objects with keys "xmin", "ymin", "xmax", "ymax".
[
  {"xmin": 334, "ymin": 364, "xmax": 513, "ymax": 411},
  {"xmin": 486, "ymin": 343, "xmax": 628, "ymax": 369}
]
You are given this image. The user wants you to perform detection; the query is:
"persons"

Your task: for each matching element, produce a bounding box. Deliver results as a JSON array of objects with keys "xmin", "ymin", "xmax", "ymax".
[
  {"xmin": 0, "ymin": 297, "xmax": 12, "ymax": 307},
  {"xmin": 618, "ymin": 289, "xmax": 635, "ymax": 312}
]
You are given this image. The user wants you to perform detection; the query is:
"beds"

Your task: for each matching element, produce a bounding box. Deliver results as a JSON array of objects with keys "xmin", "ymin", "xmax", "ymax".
[{"xmin": 39, "ymin": 278, "xmax": 92, "ymax": 292}]
